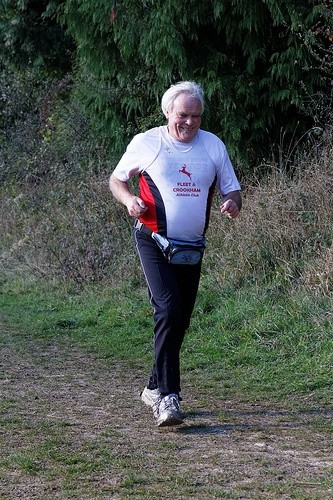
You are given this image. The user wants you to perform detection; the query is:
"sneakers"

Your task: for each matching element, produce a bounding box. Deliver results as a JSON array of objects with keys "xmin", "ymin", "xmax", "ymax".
[
  {"xmin": 141, "ymin": 386, "xmax": 162, "ymax": 419},
  {"xmin": 157, "ymin": 393, "xmax": 184, "ymax": 427}
]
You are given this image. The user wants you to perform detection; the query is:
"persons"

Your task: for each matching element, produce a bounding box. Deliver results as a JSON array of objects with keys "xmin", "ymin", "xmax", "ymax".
[{"xmin": 110, "ymin": 81, "xmax": 243, "ymax": 427}]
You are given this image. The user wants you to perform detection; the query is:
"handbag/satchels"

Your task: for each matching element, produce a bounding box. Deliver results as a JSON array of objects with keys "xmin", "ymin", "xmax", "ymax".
[{"xmin": 133, "ymin": 218, "xmax": 206, "ymax": 265}]
